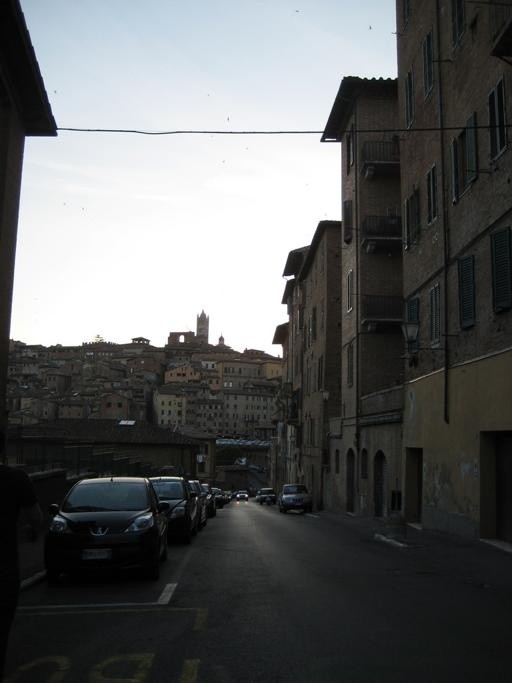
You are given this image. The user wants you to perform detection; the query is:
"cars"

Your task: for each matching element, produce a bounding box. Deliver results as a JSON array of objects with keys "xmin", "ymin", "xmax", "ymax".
[
  {"xmin": 237, "ymin": 481, "xmax": 314, "ymax": 515},
  {"xmin": 39, "ymin": 469, "xmax": 237, "ymax": 587}
]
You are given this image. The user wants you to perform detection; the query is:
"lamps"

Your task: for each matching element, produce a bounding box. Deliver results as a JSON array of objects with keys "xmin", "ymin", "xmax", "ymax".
[{"xmin": 399, "ymin": 321, "xmax": 445, "ymax": 354}]
